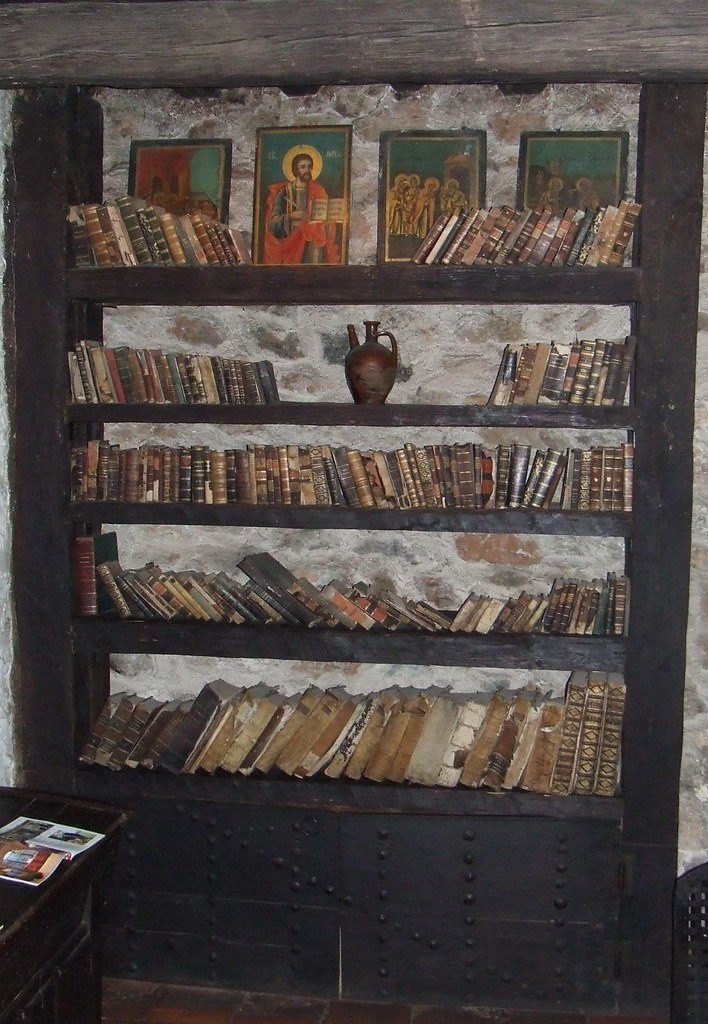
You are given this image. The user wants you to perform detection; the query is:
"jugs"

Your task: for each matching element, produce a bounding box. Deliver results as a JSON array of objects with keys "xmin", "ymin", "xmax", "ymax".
[{"xmin": 346, "ymin": 320, "xmax": 399, "ymax": 406}]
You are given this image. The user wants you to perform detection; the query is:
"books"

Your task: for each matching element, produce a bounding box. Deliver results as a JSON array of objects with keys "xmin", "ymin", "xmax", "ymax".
[
  {"xmin": 0, "ymin": 816, "xmax": 107, "ymax": 887},
  {"xmin": 64, "ymin": 177, "xmax": 642, "ymax": 801}
]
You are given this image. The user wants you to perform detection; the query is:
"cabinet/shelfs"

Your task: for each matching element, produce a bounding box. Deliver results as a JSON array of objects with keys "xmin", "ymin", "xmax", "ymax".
[
  {"xmin": 0, "ymin": 787, "xmax": 136, "ymax": 1022},
  {"xmin": 9, "ymin": 79, "xmax": 672, "ymax": 1023}
]
